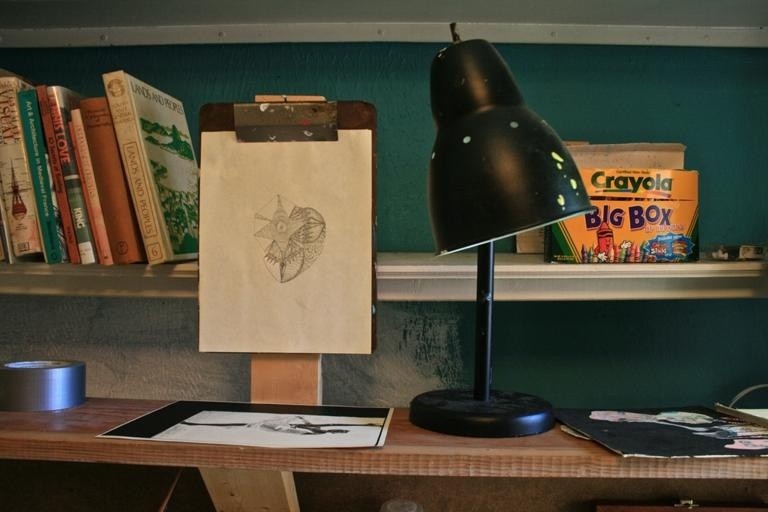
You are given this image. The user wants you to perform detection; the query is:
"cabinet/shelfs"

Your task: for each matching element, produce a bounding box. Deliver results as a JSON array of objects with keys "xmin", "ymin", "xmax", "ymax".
[{"xmin": 1, "ymin": 0, "xmax": 768, "ymax": 299}]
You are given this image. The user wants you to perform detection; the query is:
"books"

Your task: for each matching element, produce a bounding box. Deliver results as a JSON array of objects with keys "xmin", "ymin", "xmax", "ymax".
[{"xmin": 0, "ymin": 69, "xmax": 197, "ymax": 268}]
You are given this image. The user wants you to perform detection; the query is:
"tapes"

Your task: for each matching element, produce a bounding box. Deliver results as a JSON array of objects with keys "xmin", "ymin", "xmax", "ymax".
[{"xmin": 0, "ymin": 360, "xmax": 86, "ymax": 412}]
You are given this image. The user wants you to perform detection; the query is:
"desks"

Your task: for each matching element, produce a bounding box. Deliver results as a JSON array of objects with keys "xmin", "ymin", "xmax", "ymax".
[{"xmin": 0, "ymin": 397, "xmax": 766, "ymax": 512}]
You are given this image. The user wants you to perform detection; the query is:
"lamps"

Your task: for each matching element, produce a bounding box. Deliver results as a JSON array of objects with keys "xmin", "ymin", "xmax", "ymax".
[{"xmin": 407, "ymin": 22, "xmax": 598, "ymax": 437}]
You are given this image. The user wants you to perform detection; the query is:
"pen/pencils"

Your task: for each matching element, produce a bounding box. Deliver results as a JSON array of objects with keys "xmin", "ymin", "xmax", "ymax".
[{"xmin": 581, "ymin": 219, "xmax": 659, "ymax": 263}]
